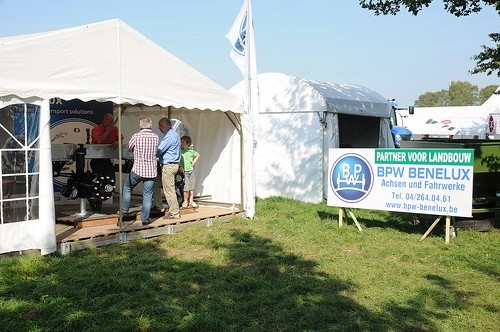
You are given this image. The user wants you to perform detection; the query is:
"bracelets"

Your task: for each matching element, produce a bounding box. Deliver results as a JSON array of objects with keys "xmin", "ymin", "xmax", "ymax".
[{"xmin": 180, "ymin": 165, "xmax": 183, "ymax": 167}]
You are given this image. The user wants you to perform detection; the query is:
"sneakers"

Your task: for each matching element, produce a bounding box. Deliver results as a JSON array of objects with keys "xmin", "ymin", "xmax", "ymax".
[
  {"xmin": 189, "ymin": 201, "xmax": 197, "ymax": 207},
  {"xmin": 182, "ymin": 201, "xmax": 188, "ymax": 208}
]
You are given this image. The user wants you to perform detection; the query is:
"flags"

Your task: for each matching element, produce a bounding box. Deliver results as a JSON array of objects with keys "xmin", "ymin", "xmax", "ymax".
[{"xmin": 225, "ymin": 0, "xmax": 258, "ymax": 89}]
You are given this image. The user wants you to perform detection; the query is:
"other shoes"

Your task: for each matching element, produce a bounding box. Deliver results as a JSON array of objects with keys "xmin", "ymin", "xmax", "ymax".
[
  {"xmin": 117, "ymin": 211, "xmax": 128, "ymax": 215},
  {"xmin": 142, "ymin": 220, "xmax": 150, "ymax": 226},
  {"xmin": 163, "ymin": 213, "xmax": 180, "ymax": 219}
]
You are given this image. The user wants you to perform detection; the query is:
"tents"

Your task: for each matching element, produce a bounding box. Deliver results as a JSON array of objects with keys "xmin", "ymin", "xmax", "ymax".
[{"xmin": 0, "ymin": 18, "xmax": 500, "ymax": 255}]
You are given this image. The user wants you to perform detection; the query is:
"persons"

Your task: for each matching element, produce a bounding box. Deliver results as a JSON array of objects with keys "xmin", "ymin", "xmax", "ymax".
[
  {"xmin": 151, "ymin": 117, "xmax": 182, "ymax": 219},
  {"xmin": 179, "ymin": 136, "xmax": 200, "ymax": 207},
  {"xmin": 89, "ymin": 112, "xmax": 123, "ymax": 210},
  {"xmin": 121, "ymin": 117, "xmax": 161, "ymax": 224}
]
